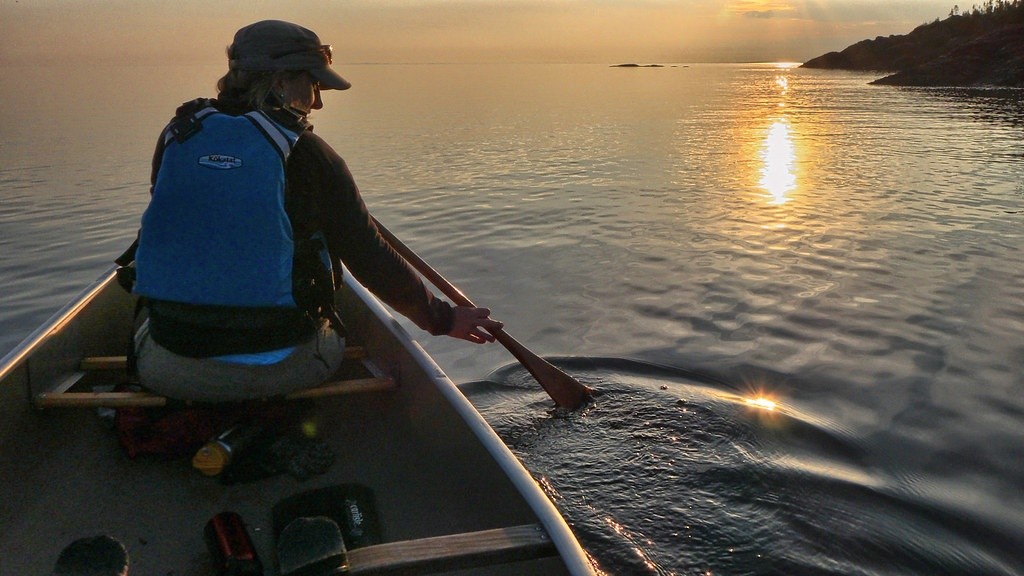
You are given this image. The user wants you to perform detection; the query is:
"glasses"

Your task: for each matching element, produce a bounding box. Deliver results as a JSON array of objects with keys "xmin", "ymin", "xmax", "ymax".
[{"xmin": 269, "ymin": 43, "xmax": 333, "ymax": 65}]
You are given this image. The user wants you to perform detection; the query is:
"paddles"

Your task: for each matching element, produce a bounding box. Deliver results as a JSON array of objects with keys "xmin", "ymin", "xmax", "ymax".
[{"xmin": 369, "ymin": 205, "xmax": 592, "ymax": 413}]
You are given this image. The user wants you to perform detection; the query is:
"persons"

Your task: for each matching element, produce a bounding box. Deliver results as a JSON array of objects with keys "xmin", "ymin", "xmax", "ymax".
[{"xmin": 116, "ymin": 20, "xmax": 505, "ymax": 398}]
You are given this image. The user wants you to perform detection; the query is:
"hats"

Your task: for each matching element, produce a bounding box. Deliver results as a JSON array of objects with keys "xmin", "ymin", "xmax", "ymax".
[{"xmin": 229, "ymin": 19, "xmax": 351, "ymax": 91}]
